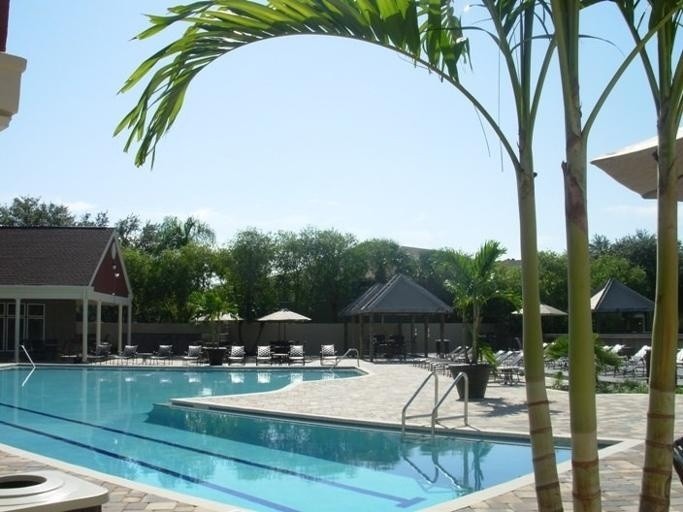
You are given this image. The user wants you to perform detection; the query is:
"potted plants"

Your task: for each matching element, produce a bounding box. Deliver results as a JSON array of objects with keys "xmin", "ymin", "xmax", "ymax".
[
  {"xmin": 186, "ymin": 286, "xmax": 244, "ymax": 368},
  {"xmin": 418, "ymin": 238, "xmax": 524, "ymax": 402}
]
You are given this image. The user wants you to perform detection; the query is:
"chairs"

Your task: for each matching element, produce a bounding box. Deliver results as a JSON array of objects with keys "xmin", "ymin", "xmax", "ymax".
[
  {"xmin": 319, "ymin": 344, "xmax": 338, "ymax": 365},
  {"xmin": 255, "ymin": 341, "xmax": 306, "ymax": 366},
  {"xmin": 412, "ymin": 343, "xmax": 682, "ymax": 384},
  {"xmin": 184, "ymin": 343, "xmax": 244, "ymax": 364},
  {"xmin": 121, "ymin": 368, "xmax": 337, "ymax": 386},
  {"xmin": 60, "ymin": 342, "xmax": 174, "ymax": 366}
]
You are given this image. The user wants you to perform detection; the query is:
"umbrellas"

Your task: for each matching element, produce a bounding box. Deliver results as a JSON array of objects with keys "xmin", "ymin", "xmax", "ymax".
[
  {"xmin": 196, "ymin": 312, "xmax": 245, "ymax": 346},
  {"xmin": 511, "ymin": 302, "xmax": 569, "ymax": 349},
  {"xmin": 256, "ymin": 308, "xmax": 313, "ymax": 347}
]
